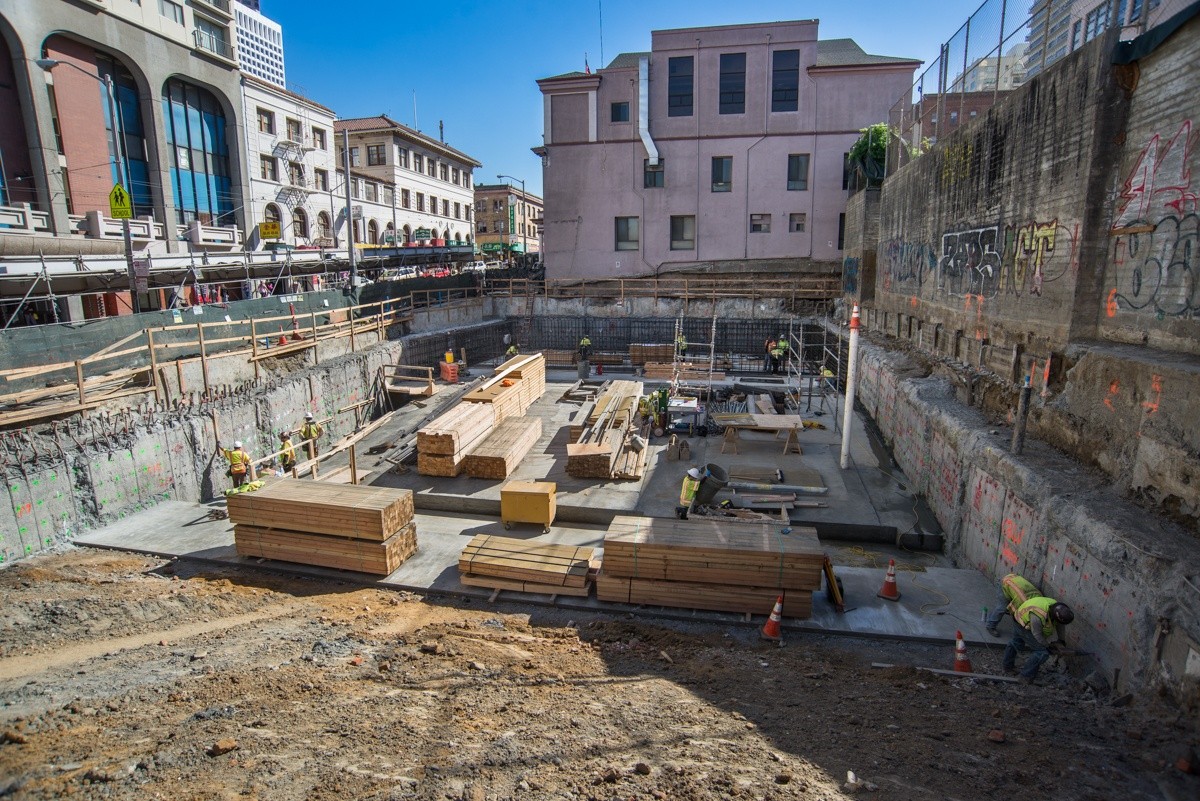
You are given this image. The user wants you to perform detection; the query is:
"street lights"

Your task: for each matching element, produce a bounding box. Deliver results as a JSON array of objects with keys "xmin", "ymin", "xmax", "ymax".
[
  {"xmin": 36, "ymin": 59, "xmax": 143, "ymax": 315},
  {"xmin": 469, "ymin": 196, "xmax": 488, "ymax": 245},
  {"xmin": 497, "ymin": 175, "xmax": 528, "ymax": 270},
  {"xmin": 330, "ymin": 177, "xmax": 356, "ymax": 249}
]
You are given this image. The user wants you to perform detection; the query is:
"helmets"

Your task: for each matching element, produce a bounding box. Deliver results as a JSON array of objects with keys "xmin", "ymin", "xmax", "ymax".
[
  {"xmin": 687, "ymin": 468, "xmax": 699, "ymax": 478},
  {"xmin": 304, "ymin": 412, "xmax": 313, "ymax": 418},
  {"xmin": 1058, "ymin": 607, "xmax": 1074, "ymax": 625},
  {"xmin": 234, "ymin": 441, "xmax": 242, "ymax": 447}
]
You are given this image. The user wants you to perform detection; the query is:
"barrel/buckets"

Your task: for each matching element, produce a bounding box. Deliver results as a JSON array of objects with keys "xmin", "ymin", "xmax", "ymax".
[{"xmin": 695, "ymin": 463, "xmax": 729, "ymax": 503}]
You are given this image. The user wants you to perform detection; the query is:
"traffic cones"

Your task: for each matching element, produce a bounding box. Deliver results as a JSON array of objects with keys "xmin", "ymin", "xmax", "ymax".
[
  {"xmin": 389, "ymin": 305, "xmax": 397, "ymax": 320},
  {"xmin": 277, "ymin": 325, "xmax": 289, "ymax": 347},
  {"xmin": 877, "ymin": 559, "xmax": 902, "ymax": 602},
  {"xmin": 759, "ymin": 594, "xmax": 785, "ymax": 643},
  {"xmin": 846, "ymin": 301, "xmax": 863, "ymax": 330},
  {"xmin": 954, "ymin": 631, "xmax": 973, "ymax": 673}
]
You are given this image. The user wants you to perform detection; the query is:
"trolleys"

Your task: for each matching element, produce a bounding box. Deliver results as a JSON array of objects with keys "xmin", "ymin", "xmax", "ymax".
[{"xmin": 500, "ymin": 482, "xmax": 557, "ymax": 534}]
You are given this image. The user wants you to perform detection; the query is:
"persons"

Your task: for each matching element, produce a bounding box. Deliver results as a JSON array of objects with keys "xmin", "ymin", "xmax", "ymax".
[
  {"xmin": 1001, "ymin": 596, "xmax": 1075, "ymax": 685},
  {"xmin": 300, "ymin": 412, "xmax": 325, "ymax": 475},
  {"xmin": 505, "ymin": 343, "xmax": 521, "ymax": 362},
  {"xmin": 243, "ymin": 280, "xmax": 268, "ymax": 299},
  {"xmin": 817, "ymin": 365, "xmax": 835, "ymax": 389},
  {"xmin": 674, "ymin": 466, "xmax": 711, "ymax": 521},
  {"xmin": 279, "ymin": 431, "xmax": 296, "ymax": 473},
  {"xmin": 23, "ymin": 302, "xmax": 38, "ymax": 325},
  {"xmin": 762, "ymin": 334, "xmax": 789, "ymax": 374},
  {"xmin": 985, "ymin": 573, "xmax": 1044, "ymax": 637},
  {"xmin": 579, "ymin": 334, "xmax": 593, "ymax": 361},
  {"xmin": 217, "ymin": 440, "xmax": 252, "ymax": 488},
  {"xmin": 678, "ymin": 332, "xmax": 688, "ymax": 362}
]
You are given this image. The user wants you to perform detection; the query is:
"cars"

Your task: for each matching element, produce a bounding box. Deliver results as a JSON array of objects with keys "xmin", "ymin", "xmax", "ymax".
[{"xmin": 343, "ymin": 257, "xmax": 521, "ymax": 293}]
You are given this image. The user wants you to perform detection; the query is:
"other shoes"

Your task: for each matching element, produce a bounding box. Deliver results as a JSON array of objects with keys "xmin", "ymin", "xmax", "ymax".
[{"xmin": 986, "ymin": 626, "xmax": 1000, "ymax": 636}]
